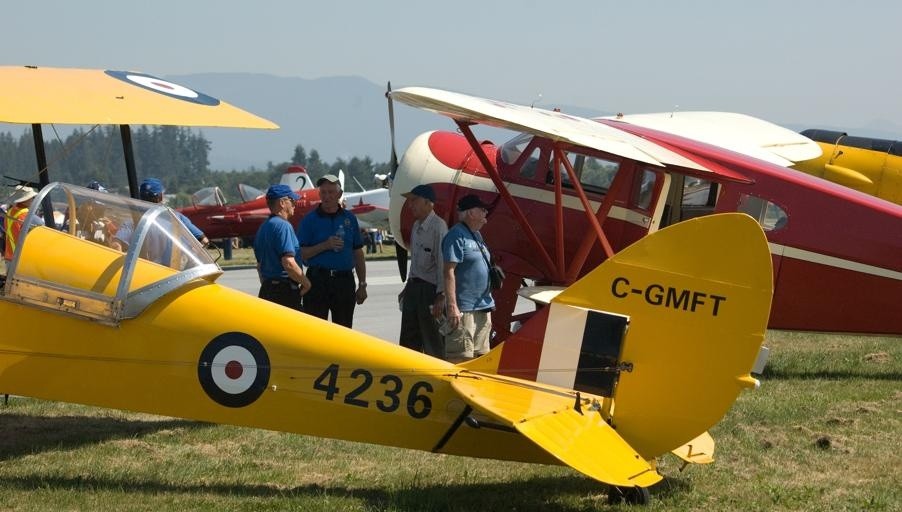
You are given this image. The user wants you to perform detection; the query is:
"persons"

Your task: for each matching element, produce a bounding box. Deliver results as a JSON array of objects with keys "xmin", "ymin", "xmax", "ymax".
[
  {"xmin": 441, "ymin": 194, "xmax": 495, "ymax": 361},
  {"xmin": 398, "ymin": 185, "xmax": 450, "ymax": 361},
  {"xmin": 298, "ymin": 174, "xmax": 367, "ymax": 329},
  {"xmin": 110, "ymin": 179, "xmax": 210, "ymax": 271},
  {"xmin": 253, "ymin": 184, "xmax": 313, "ymax": 311},
  {"xmin": 5, "ymin": 184, "xmax": 45, "ymax": 274},
  {"xmin": 62, "ymin": 180, "xmax": 120, "ymax": 248},
  {"xmin": 362, "ymin": 226, "xmax": 384, "ymax": 254}
]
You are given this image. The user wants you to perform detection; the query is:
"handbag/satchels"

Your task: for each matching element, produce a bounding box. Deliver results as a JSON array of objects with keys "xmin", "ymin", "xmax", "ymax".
[{"xmin": 488, "ymin": 263, "xmax": 506, "ymax": 290}]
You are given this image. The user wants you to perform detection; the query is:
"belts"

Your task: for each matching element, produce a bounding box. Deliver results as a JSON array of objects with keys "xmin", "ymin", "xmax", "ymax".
[{"xmin": 317, "ymin": 268, "xmax": 351, "ymax": 278}]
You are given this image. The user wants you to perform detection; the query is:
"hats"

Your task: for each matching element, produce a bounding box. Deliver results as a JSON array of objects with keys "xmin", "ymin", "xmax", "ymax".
[
  {"xmin": 10, "ymin": 185, "xmax": 39, "ymax": 204},
  {"xmin": 401, "ymin": 184, "xmax": 436, "ymax": 204},
  {"xmin": 140, "ymin": 178, "xmax": 162, "ymax": 196},
  {"xmin": 265, "ymin": 184, "xmax": 302, "ymax": 200},
  {"xmin": 457, "ymin": 194, "xmax": 494, "ymax": 211},
  {"xmin": 316, "ymin": 174, "xmax": 341, "ymax": 186}
]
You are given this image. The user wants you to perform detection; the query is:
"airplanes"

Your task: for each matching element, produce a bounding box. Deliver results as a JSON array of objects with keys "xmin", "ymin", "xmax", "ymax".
[
  {"xmin": 172, "ymin": 166, "xmax": 349, "ymax": 257},
  {"xmin": 279, "ymin": 160, "xmax": 390, "ymax": 232},
  {"xmin": 0, "ymin": 63, "xmax": 775, "ymax": 486},
  {"xmin": 378, "ymin": 80, "xmax": 901, "ymax": 354},
  {"xmin": 782, "ymin": 124, "xmax": 901, "ymax": 206}
]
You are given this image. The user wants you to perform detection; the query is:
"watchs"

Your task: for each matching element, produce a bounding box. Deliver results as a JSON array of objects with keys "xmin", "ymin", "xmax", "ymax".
[{"xmin": 360, "ymin": 282, "xmax": 367, "ymax": 289}]
